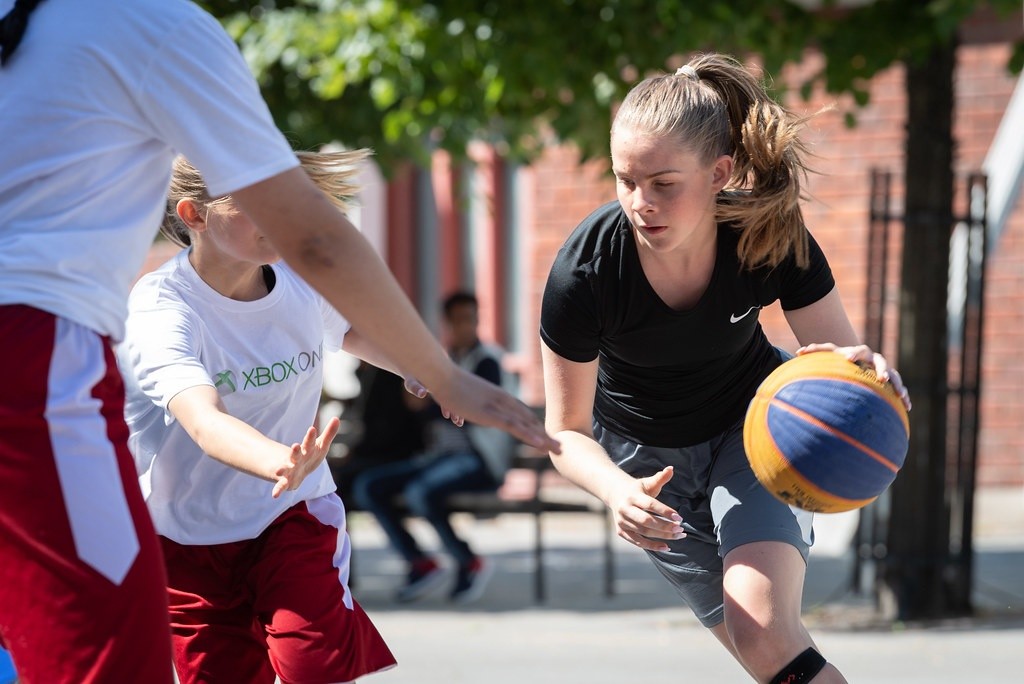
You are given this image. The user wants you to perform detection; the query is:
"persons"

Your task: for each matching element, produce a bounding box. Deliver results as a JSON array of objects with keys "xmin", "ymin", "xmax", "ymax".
[
  {"xmin": 538, "ymin": 52, "xmax": 912, "ymax": 684},
  {"xmin": 0, "ymin": 0, "xmax": 560, "ymax": 684}
]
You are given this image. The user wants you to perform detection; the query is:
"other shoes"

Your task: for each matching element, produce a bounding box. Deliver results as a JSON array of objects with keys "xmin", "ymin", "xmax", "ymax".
[
  {"xmin": 449, "ymin": 551, "xmax": 493, "ymax": 607},
  {"xmin": 396, "ymin": 554, "xmax": 443, "ymax": 602}
]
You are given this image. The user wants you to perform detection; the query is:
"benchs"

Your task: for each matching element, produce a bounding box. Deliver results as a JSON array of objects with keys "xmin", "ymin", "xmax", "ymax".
[{"xmin": 312, "ymin": 388, "xmax": 618, "ymax": 606}]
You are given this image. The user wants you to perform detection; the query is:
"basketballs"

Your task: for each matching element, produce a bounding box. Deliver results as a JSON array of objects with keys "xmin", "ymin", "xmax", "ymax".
[{"xmin": 741, "ymin": 349, "xmax": 913, "ymax": 515}]
